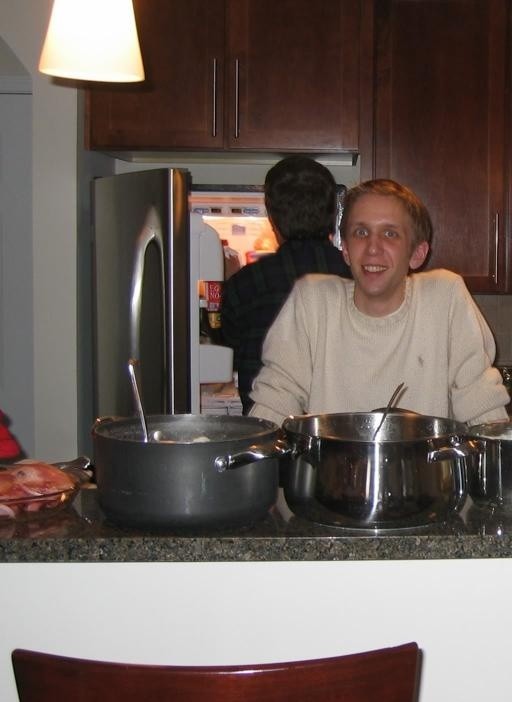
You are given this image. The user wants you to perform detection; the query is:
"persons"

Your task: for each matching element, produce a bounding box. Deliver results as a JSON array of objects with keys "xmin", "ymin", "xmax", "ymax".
[
  {"xmin": 241, "ymin": 178, "xmax": 511, "ymax": 433},
  {"xmin": 219, "ymin": 153, "xmax": 356, "ymax": 419}
]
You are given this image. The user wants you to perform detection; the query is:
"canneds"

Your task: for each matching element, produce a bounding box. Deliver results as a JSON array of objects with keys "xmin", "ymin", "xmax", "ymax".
[{"xmin": 199, "ymin": 299, "xmax": 223, "ymax": 334}]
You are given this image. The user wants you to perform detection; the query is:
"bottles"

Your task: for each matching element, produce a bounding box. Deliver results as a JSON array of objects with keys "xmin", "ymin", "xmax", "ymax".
[{"xmin": 194, "ymin": 236, "xmax": 242, "ymax": 345}]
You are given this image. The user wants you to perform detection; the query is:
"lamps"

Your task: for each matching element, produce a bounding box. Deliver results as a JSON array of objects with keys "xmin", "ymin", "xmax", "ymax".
[{"xmin": 40, "ymin": 1, "xmax": 145, "ymax": 84}]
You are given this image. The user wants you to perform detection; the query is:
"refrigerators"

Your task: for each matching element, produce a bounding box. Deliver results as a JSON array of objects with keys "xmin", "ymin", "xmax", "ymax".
[{"xmin": 87, "ymin": 165, "xmax": 351, "ymax": 428}]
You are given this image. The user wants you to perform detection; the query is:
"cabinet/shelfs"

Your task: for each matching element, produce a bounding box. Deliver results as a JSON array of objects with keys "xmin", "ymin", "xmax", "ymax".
[
  {"xmin": 86, "ymin": 2, "xmax": 360, "ymax": 156},
  {"xmin": 369, "ymin": 2, "xmax": 511, "ymax": 295}
]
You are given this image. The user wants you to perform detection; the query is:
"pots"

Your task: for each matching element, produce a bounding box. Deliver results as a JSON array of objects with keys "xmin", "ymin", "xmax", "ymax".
[
  {"xmin": 282, "ymin": 407, "xmax": 469, "ymax": 527},
  {"xmin": 464, "ymin": 422, "xmax": 512, "ymax": 513},
  {"xmin": 91, "ymin": 414, "xmax": 290, "ymax": 532}
]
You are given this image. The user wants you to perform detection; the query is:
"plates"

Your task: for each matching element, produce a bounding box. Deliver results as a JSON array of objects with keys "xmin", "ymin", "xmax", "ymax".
[{"xmin": 0, "ymin": 454, "xmax": 96, "ymax": 528}]
